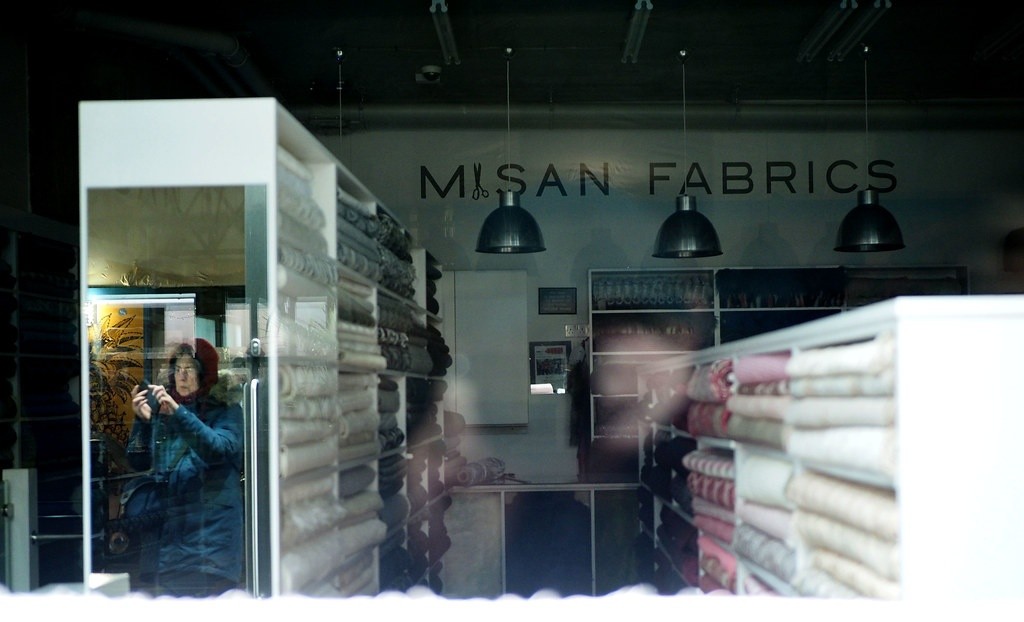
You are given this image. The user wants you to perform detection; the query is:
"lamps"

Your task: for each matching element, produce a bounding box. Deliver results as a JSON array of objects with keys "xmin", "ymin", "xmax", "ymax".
[
  {"xmin": 475, "ymin": 48, "xmax": 546, "ymax": 255},
  {"xmin": 828, "ymin": 0, "xmax": 892, "ymax": 63},
  {"xmin": 621, "ymin": 0, "xmax": 653, "ymax": 63},
  {"xmin": 973, "ymin": 22, "xmax": 1024, "ymax": 64},
  {"xmin": 430, "ymin": 0, "xmax": 461, "ymax": 66},
  {"xmin": 652, "ymin": 49, "xmax": 723, "ymax": 259},
  {"xmin": 796, "ymin": 0, "xmax": 858, "ymax": 64},
  {"xmin": 834, "ymin": 47, "xmax": 905, "ymax": 253}
]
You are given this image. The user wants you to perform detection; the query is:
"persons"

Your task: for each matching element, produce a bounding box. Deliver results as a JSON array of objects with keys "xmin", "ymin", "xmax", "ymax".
[{"xmin": 127, "ymin": 338, "xmax": 244, "ymax": 596}]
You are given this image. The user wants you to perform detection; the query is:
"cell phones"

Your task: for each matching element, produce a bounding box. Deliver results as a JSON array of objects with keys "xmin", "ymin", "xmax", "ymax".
[{"xmin": 140, "ymin": 379, "xmax": 161, "ymax": 417}]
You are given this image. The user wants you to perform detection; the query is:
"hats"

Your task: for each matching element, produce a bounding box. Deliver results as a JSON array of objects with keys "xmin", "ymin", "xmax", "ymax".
[{"xmin": 169, "ymin": 338, "xmax": 219, "ymax": 385}]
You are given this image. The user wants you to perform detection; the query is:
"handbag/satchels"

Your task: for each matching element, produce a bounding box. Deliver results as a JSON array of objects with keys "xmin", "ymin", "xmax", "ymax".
[{"xmin": 115, "ymin": 474, "xmax": 172, "ymax": 532}]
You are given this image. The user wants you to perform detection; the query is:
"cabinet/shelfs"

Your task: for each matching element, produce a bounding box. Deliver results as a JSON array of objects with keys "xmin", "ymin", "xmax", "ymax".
[
  {"xmin": 635, "ymin": 295, "xmax": 1024, "ymax": 596},
  {"xmin": 588, "ymin": 264, "xmax": 970, "ymax": 442},
  {"xmin": 0, "ymin": 203, "xmax": 82, "ymax": 484},
  {"xmin": 79, "ymin": 97, "xmax": 445, "ymax": 598},
  {"xmin": 443, "ymin": 270, "xmax": 530, "ymax": 428},
  {"xmin": 445, "ymin": 483, "xmax": 638, "ymax": 599}
]
